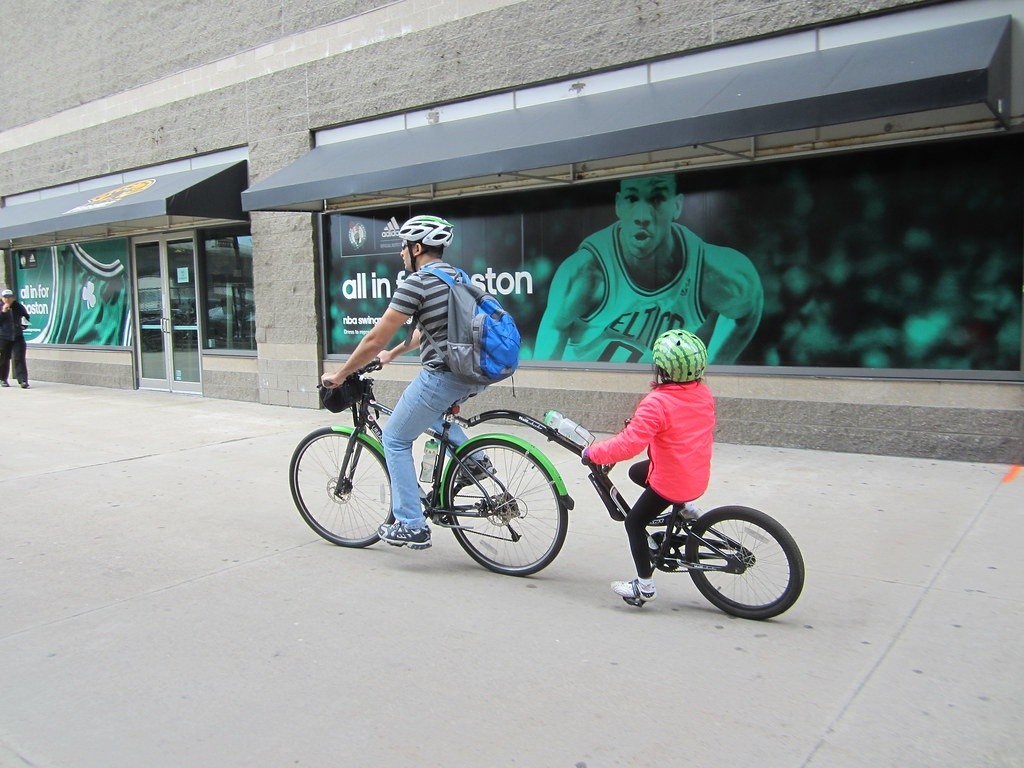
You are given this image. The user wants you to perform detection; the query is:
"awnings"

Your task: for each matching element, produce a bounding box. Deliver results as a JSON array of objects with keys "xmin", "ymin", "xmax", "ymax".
[
  {"xmin": 1, "ymin": 159, "xmax": 247, "ymax": 251},
  {"xmin": 241, "ymin": 13, "xmax": 1013, "ymax": 217}
]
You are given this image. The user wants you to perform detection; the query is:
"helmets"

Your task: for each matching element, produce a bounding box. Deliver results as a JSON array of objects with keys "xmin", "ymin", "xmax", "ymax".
[
  {"xmin": 652, "ymin": 329, "xmax": 707, "ymax": 382},
  {"xmin": 398, "ymin": 215, "xmax": 454, "ymax": 246}
]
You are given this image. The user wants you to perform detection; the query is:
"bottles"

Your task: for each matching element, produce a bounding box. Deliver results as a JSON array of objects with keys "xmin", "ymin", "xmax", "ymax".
[
  {"xmin": 542, "ymin": 409, "xmax": 592, "ymax": 449},
  {"xmin": 420, "ymin": 437, "xmax": 439, "ymax": 482}
]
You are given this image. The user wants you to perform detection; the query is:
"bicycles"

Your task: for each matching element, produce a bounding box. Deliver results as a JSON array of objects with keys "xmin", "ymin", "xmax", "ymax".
[{"xmin": 288, "ymin": 372, "xmax": 805, "ymax": 622}]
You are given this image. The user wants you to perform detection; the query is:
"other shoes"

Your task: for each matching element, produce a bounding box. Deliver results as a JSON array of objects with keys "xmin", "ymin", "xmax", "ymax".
[
  {"xmin": 21, "ymin": 382, "xmax": 29, "ymax": 389},
  {"xmin": 0, "ymin": 380, "xmax": 9, "ymax": 387}
]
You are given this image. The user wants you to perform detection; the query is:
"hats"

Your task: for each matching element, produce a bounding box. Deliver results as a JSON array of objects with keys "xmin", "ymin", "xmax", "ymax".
[{"xmin": 2, "ymin": 289, "xmax": 13, "ymax": 297}]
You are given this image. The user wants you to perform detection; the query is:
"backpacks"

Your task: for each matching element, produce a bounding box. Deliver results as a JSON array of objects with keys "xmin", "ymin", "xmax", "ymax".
[{"xmin": 415, "ymin": 267, "xmax": 521, "ymax": 384}]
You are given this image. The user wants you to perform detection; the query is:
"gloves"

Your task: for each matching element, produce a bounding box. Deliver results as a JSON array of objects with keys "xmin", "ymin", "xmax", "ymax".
[{"xmin": 582, "ymin": 447, "xmax": 591, "ymax": 460}]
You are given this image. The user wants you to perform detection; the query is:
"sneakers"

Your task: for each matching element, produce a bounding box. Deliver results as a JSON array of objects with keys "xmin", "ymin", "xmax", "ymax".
[
  {"xmin": 674, "ymin": 502, "xmax": 704, "ymax": 529},
  {"xmin": 454, "ymin": 455, "xmax": 498, "ymax": 484},
  {"xmin": 378, "ymin": 521, "xmax": 433, "ymax": 550},
  {"xmin": 611, "ymin": 578, "xmax": 657, "ymax": 602}
]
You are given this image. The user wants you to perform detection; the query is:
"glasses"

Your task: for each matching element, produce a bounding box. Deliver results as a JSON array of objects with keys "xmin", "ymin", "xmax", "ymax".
[{"xmin": 401, "ymin": 243, "xmax": 425, "ymax": 250}]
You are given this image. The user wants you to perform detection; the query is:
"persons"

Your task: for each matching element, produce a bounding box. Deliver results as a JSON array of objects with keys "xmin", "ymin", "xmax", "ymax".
[
  {"xmin": 581, "ymin": 329, "xmax": 716, "ymax": 603},
  {"xmin": 0, "ymin": 290, "xmax": 32, "ymax": 389},
  {"xmin": 529, "ymin": 170, "xmax": 764, "ymax": 368},
  {"xmin": 322, "ymin": 216, "xmax": 497, "ymax": 550}
]
